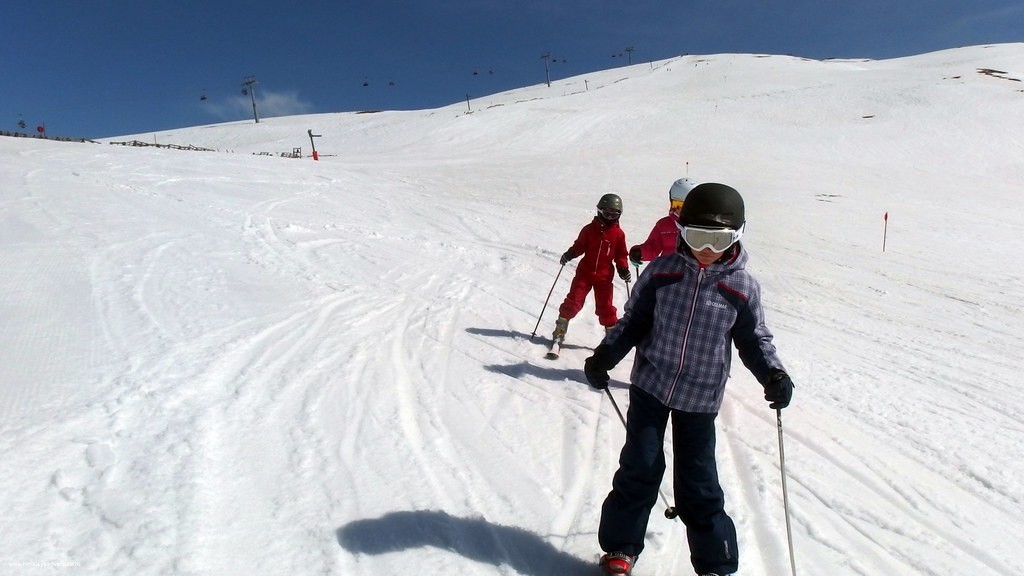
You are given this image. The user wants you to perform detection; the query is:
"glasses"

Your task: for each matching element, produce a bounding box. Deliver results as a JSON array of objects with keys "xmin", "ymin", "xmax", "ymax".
[
  {"xmin": 597, "ymin": 207, "xmax": 621, "ymax": 219},
  {"xmin": 675, "ymin": 221, "xmax": 744, "ymax": 253},
  {"xmin": 672, "ymin": 200, "xmax": 684, "ymax": 208}
]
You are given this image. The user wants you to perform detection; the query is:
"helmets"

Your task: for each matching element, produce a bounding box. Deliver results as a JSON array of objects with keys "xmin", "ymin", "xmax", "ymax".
[
  {"xmin": 596, "ymin": 194, "xmax": 623, "ymax": 212},
  {"xmin": 669, "ymin": 178, "xmax": 700, "ymax": 201},
  {"xmin": 677, "ymin": 182, "xmax": 744, "ymax": 229}
]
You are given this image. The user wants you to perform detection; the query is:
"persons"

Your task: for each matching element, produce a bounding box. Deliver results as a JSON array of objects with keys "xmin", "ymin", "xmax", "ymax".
[
  {"xmin": 628, "ymin": 178, "xmax": 697, "ymax": 267},
  {"xmin": 551, "ymin": 194, "xmax": 632, "ymax": 347},
  {"xmin": 584, "ymin": 183, "xmax": 793, "ymax": 576}
]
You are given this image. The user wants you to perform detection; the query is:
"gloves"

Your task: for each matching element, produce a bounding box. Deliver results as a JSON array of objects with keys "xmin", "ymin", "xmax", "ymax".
[
  {"xmin": 560, "ymin": 247, "xmax": 577, "ymax": 266},
  {"xmin": 765, "ymin": 370, "xmax": 794, "ymax": 409},
  {"xmin": 618, "ymin": 269, "xmax": 631, "ymax": 282},
  {"xmin": 629, "ymin": 246, "xmax": 643, "ymax": 268},
  {"xmin": 585, "ymin": 350, "xmax": 615, "ymax": 390}
]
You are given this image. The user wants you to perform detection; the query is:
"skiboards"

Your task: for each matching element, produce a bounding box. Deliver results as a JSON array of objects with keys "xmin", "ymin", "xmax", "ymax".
[{"xmin": 545, "ymin": 322, "xmax": 566, "ymax": 363}]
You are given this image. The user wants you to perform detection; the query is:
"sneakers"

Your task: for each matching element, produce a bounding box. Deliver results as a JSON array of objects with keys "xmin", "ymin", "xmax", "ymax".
[
  {"xmin": 596, "ymin": 551, "xmax": 635, "ymax": 576},
  {"xmin": 553, "ymin": 316, "xmax": 569, "ymax": 343},
  {"xmin": 605, "ymin": 326, "xmax": 615, "ymax": 335}
]
